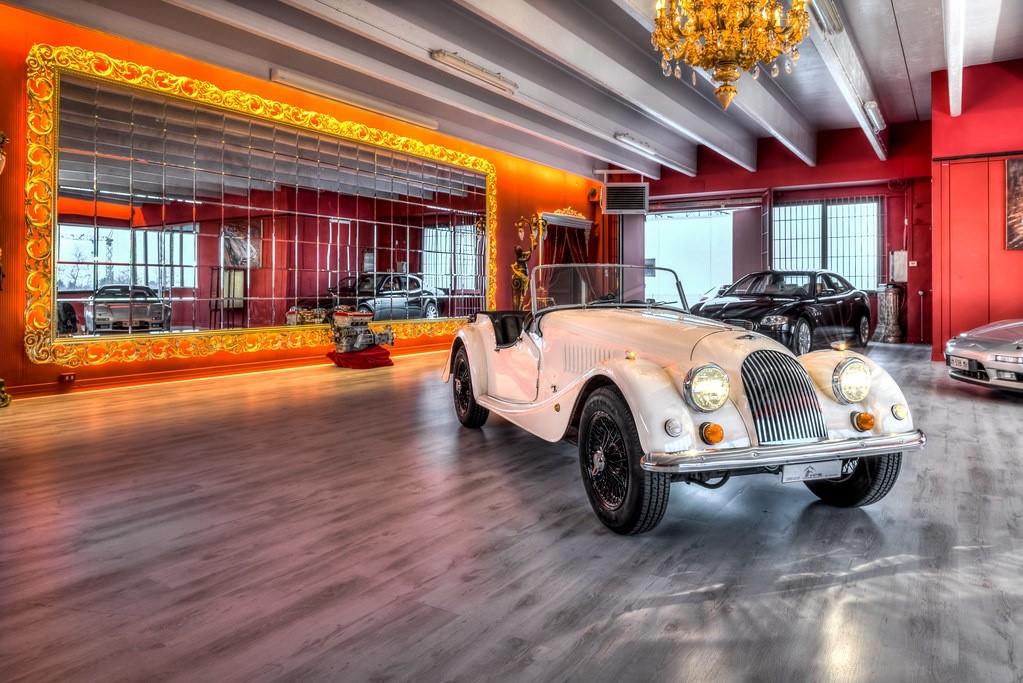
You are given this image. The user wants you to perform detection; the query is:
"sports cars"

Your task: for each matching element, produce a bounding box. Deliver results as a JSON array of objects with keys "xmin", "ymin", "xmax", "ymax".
[
  {"xmin": 943, "ymin": 317, "xmax": 1022, "ymax": 395},
  {"xmin": 85, "ymin": 286, "xmax": 171, "ymax": 334}
]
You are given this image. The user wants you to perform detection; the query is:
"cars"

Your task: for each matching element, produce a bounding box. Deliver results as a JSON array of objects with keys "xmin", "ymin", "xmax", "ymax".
[
  {"xmin": 689, "ymin": 269, "xmax": 872, "ymax": 356},
  {"xmin": 441, "ymin": 262, "xmax": 925, "ymax": 536},
  {"xmin": 298, "ymin": 276, "xmax": 445, "ymax": 322}
]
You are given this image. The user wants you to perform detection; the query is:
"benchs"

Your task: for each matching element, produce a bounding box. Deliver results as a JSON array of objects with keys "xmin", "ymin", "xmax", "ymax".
[{"xmin": 474, "ymin": 310, "xmax": 531, "ymax": 322}]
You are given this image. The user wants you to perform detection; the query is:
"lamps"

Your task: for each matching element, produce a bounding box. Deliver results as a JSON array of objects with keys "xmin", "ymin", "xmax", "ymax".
[
  {"xmin": 270, "ymin": 66, "xmax": 438, "ymax": 131},
  {"xmin": 864, "ymin": 101, "xmax": 886, "ymax": 134},
  {"xmin": 811, "ymin": 0, "xmax": 845, "ymax": 36},
  {"xmin": 433, "ymin": 49, "xmax": 520, "ymax": 94},
  {"xmin": 650, "ymin": 0, "xmax": 810, "ymax": 111},
  {"xmin": 614, "ymin": 134, "xmax": 656, "ymax": 156}
]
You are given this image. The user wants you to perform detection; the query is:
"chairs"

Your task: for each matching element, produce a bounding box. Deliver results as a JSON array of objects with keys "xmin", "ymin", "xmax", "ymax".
[
  {"xmin": 764, "ymin": 284, "xmax": 781, "ymax": 295},
  {"xmin": 804, "ymin": 283, "xmax": 823, "ymax": 296},
  {"xmin": 495, "ymin": 315, "xmax": 524, "ymax": 344},
  {"xmin": 132, "ymin": 292, "xmax": 147, "ymax": 298}
]
